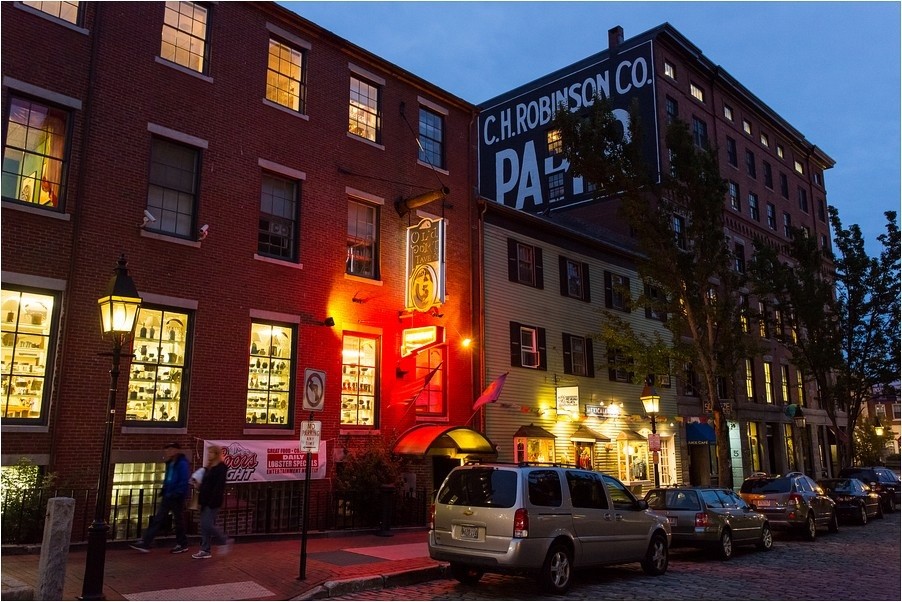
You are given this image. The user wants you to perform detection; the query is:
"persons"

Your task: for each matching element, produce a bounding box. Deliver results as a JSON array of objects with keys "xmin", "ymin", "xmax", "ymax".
[
  {"xmin": 129, "ymin": 443, "xmax": 193, "ymax": 554},
  {"xmin": 188, "ymin": 446, "xmax": 235, "ymax": 558}
]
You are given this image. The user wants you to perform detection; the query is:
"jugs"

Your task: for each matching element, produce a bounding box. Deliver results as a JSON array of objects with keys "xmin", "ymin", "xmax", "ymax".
[
  {"xmin": 138, "ymin": 344, "xmax": 147, "ymax": 360},
  {"xmin": 30, "ymin": 311, "xmax": 46, "ymax": 325}
]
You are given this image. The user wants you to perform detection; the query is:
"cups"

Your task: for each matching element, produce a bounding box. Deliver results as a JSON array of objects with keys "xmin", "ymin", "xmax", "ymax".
[{"xmin": 158, "ymin": 355, "xmax": 164, "ymax": 363}]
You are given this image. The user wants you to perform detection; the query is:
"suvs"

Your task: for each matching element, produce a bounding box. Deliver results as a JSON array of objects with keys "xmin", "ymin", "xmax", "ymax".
[
  {"xmin": 837, "ymin": 466, "xmax": 901, "ymax": 514},
  {"xmin": 738, "ymin": 472, "xmax": 839, "ymax": 541},
  {"xmin": 642, "ymin": 484, "xmax": 774, "ymax": 562}
]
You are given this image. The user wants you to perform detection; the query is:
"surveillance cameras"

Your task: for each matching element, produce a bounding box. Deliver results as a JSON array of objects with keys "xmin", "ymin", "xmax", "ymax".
[
  {"xmin": 198, "ymin": 224, "xmax": 209, "ymax": 235},
  {"xmin": 144, "ymin": 210, "xmax": 156, "ymax": 223}
]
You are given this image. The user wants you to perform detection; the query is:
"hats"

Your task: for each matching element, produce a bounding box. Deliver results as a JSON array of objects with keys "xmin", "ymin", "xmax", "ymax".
[{"xmin": 164, "ymin": 442, "xmax": 178, "ymax": 449}]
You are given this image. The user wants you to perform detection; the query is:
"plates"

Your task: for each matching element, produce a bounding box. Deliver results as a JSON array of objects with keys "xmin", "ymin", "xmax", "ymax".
[{"xmin": 2, "ymin": 333, "xmax": 19, "ymax": 347}]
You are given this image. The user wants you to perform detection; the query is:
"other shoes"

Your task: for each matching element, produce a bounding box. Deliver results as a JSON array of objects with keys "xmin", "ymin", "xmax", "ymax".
[
  {"xmin": 171, "ymin": 545, "xmax": 188, "ymax": 553},
  {"xmin": 192, "ymin": 550, "xmax": 212, "ymax": 558},
  {"xmin": 130, "ymin": 543, "xmax": 150, "ymax": 553},
  {"xmin": 218, "ymin": 545, "xmax": 227, "ymax": 554}
]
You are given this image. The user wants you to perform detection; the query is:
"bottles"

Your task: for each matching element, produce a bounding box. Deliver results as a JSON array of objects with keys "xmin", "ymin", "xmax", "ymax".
[
  {"xmin": 140, "ymin": 323, "xmax": 175, "ymax": 340},
  {"xmin": 256, "ymin": 358, "xmax": 260, "ymax": 368}
]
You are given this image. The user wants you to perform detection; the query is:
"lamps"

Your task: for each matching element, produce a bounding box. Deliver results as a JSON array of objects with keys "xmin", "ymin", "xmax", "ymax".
[{"xmin": 319, "ymin": 317, "xmax": 335, "ymax": 327}]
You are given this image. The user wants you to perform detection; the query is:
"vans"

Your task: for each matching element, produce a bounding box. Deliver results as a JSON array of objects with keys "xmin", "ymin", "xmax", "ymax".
[{"xmin": 428, "ymin": 460, "xmax": 660, "ymax": 594}]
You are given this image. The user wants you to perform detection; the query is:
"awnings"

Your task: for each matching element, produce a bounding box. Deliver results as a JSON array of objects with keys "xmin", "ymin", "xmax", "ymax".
[{"xmin": 686, "ymin": 421, "xmax": 719, "ymax": 445}]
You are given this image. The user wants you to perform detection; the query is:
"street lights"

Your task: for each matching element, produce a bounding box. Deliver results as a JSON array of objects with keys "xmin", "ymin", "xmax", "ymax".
[
  {"xmin": 640, "ymin": 379, "xmax": 661, "ymax": 488},
  {"xmin": 875, "ymin": 419, "xmax": 885, "ymax": 468},
  {"xmin": 793, "ymin": 404, "xmax": 807, "ymax": 476},
  {"xmin": 78, "ymin": 253, "xmax": 144, "ymax": 601}
]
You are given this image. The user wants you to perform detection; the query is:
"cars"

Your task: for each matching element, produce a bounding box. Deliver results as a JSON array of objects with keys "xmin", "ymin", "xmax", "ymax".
[{"xmin": 815, "ymin": 478, "xmax": 884, "ymax": 525}]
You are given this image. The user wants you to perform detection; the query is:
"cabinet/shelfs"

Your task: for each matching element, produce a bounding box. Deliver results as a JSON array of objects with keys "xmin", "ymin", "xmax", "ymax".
[
  {"xmin": 249, "ymin": 354, "xmax": 291, "ymax": 427},
  {"xmin": 1, "ymin": 321, "xmax": 50, "ymax": 416},
  {"xmin": 125, "ymin": 337, "xmax": 185, "ymax": 422},
  {"xmin": 340, "ymin": 363, "xmax": 376, "ymax": 425}
]
